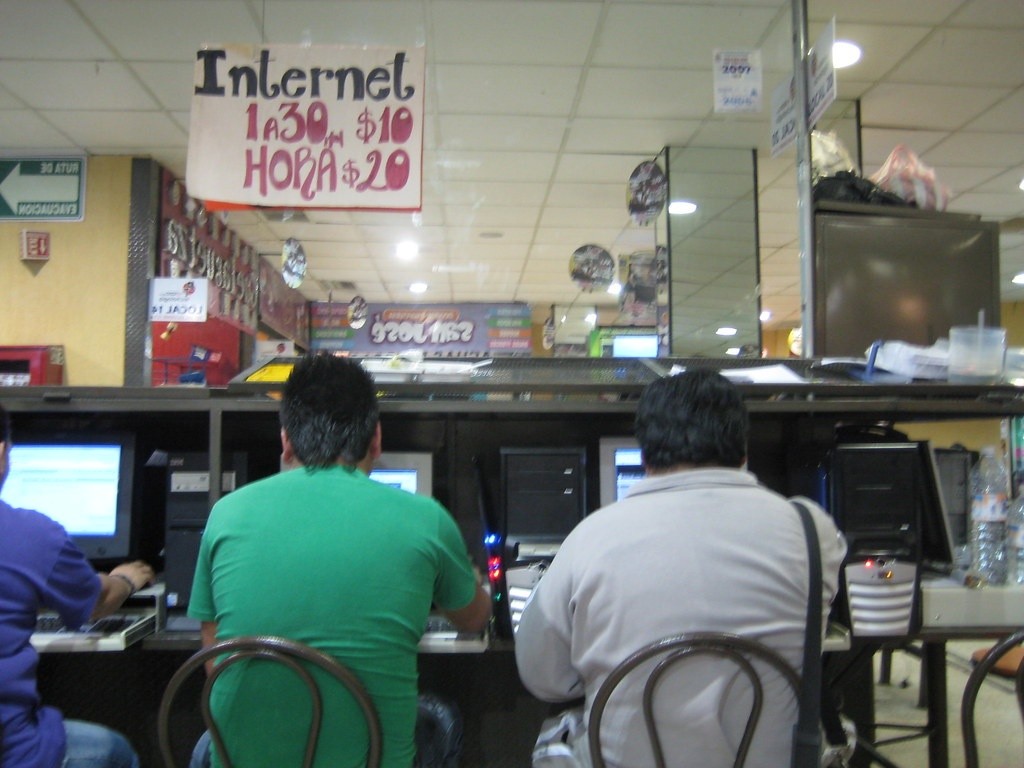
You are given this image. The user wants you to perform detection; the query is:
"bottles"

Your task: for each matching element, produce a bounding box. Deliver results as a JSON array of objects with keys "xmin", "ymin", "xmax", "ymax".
[
  {"xmin": 966, "ymin": 444, "xmax": 1010, "ymax": 586},
  {"xmin": 1008, "ymin": 483, "xmax": 1024, "ymax": 585}
]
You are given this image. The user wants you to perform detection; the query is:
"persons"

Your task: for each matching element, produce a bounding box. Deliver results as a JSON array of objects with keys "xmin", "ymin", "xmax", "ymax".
[
  {"xmin": 187, "ymin": 351, "xmax": 492, "ymax": 768},
  {"xmin": 0, "ymin": 403, "xmax": 154, "ymax": 768},
  {"xmin": 514, "ymin": 369, "xmax": 848, "ymax": 768}
]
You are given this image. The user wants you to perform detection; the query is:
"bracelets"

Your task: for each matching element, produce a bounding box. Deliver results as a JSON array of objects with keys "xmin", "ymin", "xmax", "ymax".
[{"xmin": 110, "ymin": 574, "xmax": 136, "ymax": 596}]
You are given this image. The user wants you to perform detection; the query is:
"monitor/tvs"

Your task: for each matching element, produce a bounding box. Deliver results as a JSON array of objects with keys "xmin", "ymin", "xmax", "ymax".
[
  {"xmin": 282, "ymin": 450, "xmax": 436, "ymax": 501},
  {"xmin": 601, "ymin": 437, "xmax": 647, "ymax": 509},
  {"xmin": 0, "ymin": 431, "xmax": 135, "ymax": 558},
  {"xmin": 915, "ymin": 439, "xmax": 975, "ymax": 573}
]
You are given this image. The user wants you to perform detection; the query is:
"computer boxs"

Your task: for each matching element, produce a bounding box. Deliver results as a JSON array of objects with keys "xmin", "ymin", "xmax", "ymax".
[
  {"xmin": 495, "ymin": 446, "xmax": 588, "ymax": 638},
  {"xmin": 163, "ymin": 448, "xmax": 250, "ymax": 630},
  {"xmin": 776, "ymin": 439, "xmax": 924, "ymax": 648}
]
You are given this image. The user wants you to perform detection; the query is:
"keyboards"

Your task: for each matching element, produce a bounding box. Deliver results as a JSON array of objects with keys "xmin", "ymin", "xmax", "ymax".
[
  {"xmin": 416, "ymin": 613, "xmax": 489, "ymax": 654},
  {"xmin": 26, "ymin": 610, "xmax": 145, "ymax": 639}
]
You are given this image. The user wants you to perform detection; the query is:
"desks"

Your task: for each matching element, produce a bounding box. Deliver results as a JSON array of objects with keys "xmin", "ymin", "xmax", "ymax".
[{"xmin": 0, "ymin": 356, "xmax": 1024, "ymax": 652}]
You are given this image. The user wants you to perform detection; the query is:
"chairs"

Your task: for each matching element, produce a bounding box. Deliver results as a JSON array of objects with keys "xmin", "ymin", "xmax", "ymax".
[
  {"xmin": 588, "ymin": 631, "xmax": 801, "ymax": 767},
  {"xmin": 156, "ymin": 639, "xmax": 383, "ymax": 768},
  {"xmin": 961, "ymin": 634, "xmax": 1024, "ymax": 768}
]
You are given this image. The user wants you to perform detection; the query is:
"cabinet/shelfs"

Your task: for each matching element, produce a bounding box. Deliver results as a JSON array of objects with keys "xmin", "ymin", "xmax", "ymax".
[{"xmin": 814, "ymin": 211, "xmax": 1000, "ymax": 360}]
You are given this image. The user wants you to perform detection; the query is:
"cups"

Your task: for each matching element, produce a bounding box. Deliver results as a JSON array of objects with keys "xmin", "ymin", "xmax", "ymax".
[{"xmin": 947, "ymin": 326, "xmax": 1008, "ymax": 385}]
